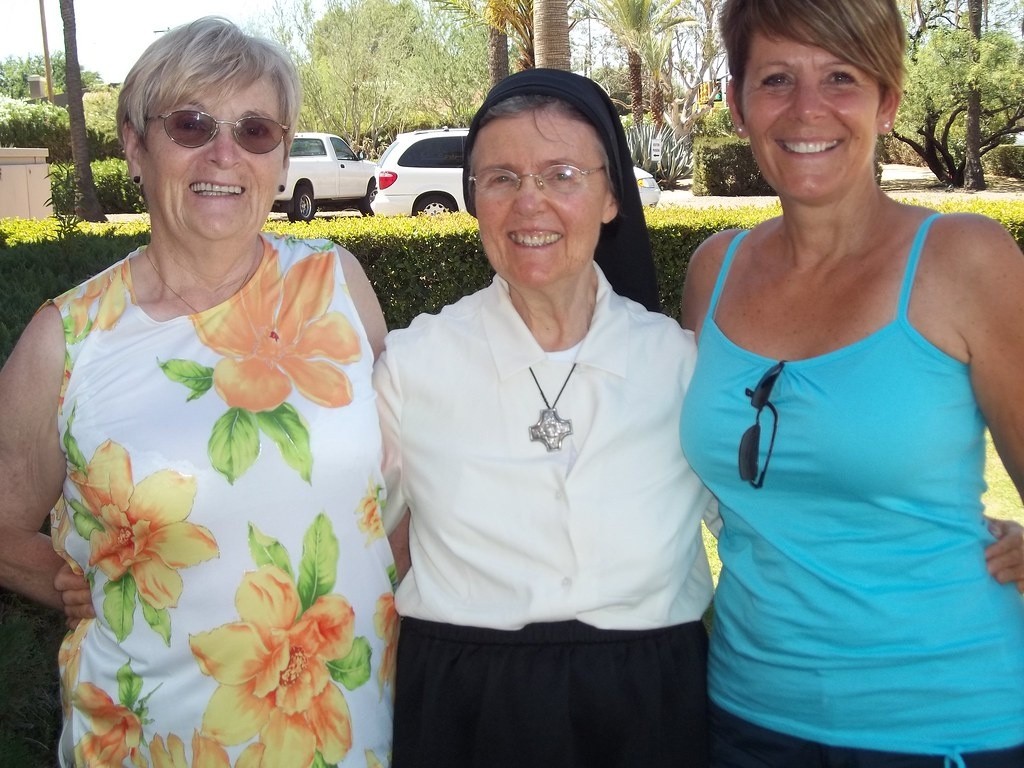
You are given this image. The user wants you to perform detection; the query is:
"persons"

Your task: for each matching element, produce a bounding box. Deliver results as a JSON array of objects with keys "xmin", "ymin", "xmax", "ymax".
[
  {"xmin": 55, "ymin": 70, "xmax": 1023, "ymax": 767},
  {"xmin": 684, "ymin": 1, "xmax": 1024, "ymax": 768},
  {"xmin": 0, "ymin": 15, "xmax": 413, "ymax": 768}
]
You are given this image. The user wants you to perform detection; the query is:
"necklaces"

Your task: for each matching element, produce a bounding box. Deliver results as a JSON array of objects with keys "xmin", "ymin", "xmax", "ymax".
[
  {"xmin": 141, "ymin": 249, "xmax": 258, "ymax": 310},
  {"xmin": 524, "ymin": 365, "xmax": 579, "ymax": 452}
]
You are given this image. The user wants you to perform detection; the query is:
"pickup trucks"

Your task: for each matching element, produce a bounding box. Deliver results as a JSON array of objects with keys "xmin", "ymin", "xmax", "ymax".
[{"xmin": 270, "ymin": 133, "xmax": 380, "ymax": 224}]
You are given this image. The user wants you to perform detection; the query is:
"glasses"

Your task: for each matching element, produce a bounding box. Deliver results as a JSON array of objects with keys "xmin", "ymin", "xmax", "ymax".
[
  {"xmin": 465, "ymin": 163, "xmax": 607, "ymax": 201},
  {"xmin": 144, "ymin": 109, "xmax": 290, "ymax": 155},
  {"xmin": 736, "ymin": 361, "xmax": 787, "ymax": 492}
]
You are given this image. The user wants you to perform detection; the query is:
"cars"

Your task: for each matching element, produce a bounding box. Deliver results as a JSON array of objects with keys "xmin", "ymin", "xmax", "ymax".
[{"xmin": 369, "ymin": 126, "xmax": 660, "ymax": 217}]
